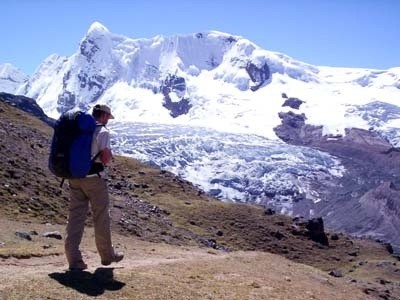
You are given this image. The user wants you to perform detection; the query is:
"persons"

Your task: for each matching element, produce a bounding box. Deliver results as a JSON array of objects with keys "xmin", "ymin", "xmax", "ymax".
[{"xmin": 62, "ymin": 103, "xmax": 125, "ymax": 271}]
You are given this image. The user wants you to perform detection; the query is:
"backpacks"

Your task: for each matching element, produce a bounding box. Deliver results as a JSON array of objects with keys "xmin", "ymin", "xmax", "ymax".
[{"xmin": 49, "ymin": 110, "xmax": 107, "ymax": 188}]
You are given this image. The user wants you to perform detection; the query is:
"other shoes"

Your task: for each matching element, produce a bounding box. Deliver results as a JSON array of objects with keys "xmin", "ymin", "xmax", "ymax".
[
  {"xmin": 102, "ymin": 251, "xmax": 124, "ymax": 266},
  {"xmin": 69, "ymin": 260, "xmax": 88, "ymax": 269}
]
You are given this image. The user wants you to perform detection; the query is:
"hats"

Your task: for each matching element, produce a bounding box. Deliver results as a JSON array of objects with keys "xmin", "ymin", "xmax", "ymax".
[{"xmin": 94, "ymin": 104, "xmax": 114, "ymax": 119}]
